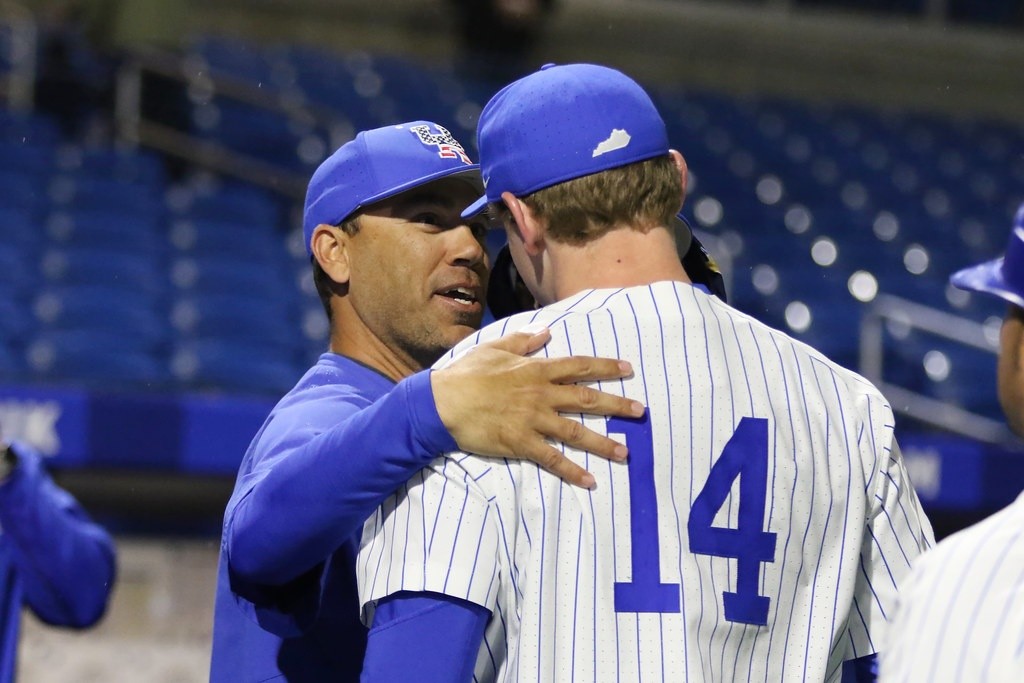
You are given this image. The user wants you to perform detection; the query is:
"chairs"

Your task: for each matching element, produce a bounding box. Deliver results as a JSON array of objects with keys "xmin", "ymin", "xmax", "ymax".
[{"xmin": 0, "ymin": 15, "xmax": 1024, "ymax": 540}]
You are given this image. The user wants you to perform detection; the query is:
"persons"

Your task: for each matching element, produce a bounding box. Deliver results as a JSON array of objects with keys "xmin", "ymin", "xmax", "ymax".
[
  {"xmin": 208, "ymin": 121, "xmax": 645, "ymax": 683},
  {"xmin": 877, "ymin": 205, "xmax": 1024, "ymax": 683},
  {"xmin": 353, "ymin": 63, "xmax": 938, "ymax": 683},
  {"xmin": 0, "ymin": 440, "xmax": 117, "ymax": 682}
]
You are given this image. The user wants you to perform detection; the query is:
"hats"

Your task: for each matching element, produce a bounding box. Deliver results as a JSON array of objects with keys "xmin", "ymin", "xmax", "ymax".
[
  {"xmin": 303, "ymin": 119, "xmax": 483, "ymax": 261},
  {"xmin": 461, "ymin": 61, "xmax": 669, "ymax": 222},
  {"xmin": 949, "ymin": 203, "xmax": 1024, "ymax": 309}
]
